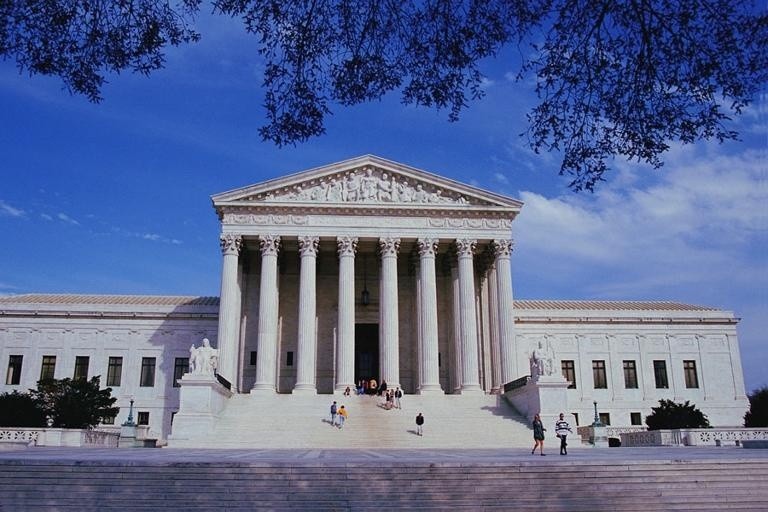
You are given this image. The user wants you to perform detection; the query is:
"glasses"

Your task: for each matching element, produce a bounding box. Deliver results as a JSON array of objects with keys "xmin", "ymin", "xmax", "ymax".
[{"xmin": 360, "ymin": 253, "xmax": 371, "ymax": 306}]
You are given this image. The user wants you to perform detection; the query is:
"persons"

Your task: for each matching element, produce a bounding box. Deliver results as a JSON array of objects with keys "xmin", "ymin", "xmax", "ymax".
[
  {"xmin": 531, "ymin": 413, "xmax": 548, "ymax": 456},
  {"xmin": 532, "ymin": 339, "xmax": 550, "ymax": 375},
  {"xmin": 189, "ymin": 338, "xmax": 218, "ymax": 377},
  {"xmin": 354, "ymin": 375, "xmax": 405, "ymax": 409},
  {"xmin": 263, "ymin": 166, "xmax": 470, "ymax": 205},
  {"xmin": 344, "ymin": 386, "xmax": 352, "ymax": 397},
  {"xmin": 330, "ymin": 400, "xmax": 338, "ymax": 425},
  {"xmin": 337, "ymin": 404, "xmax": 349, "ymax": 430},
  {"xmin": 555, "ymin": 412, "xmax": 574, "ymax": 456},
  {"xmin": 415, "ymin": 412, "xmax": 424, "ymax": 437}
]
